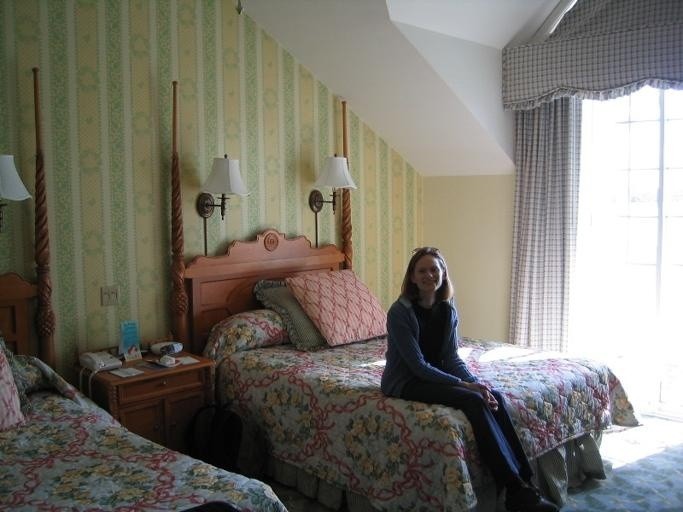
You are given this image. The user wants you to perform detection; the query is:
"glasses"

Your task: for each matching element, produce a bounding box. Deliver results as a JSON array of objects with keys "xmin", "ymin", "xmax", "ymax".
[{"xmin": 409, "ymin": 246, "xmax": 442, "ymax": 255}]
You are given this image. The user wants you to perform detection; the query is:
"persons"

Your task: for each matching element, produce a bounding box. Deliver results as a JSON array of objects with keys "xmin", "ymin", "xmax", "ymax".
[{"xmin": 380, "ymin": 246, "xmax": 558, "ymax": 512}]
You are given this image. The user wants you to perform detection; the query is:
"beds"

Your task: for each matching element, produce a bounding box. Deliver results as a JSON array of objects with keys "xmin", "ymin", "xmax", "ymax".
[
  {"xmin": 0, "ymin": 272, "xmax": 286, "ymax": 512},
  {"xmin": 179, "ymin": 227, "xmax": 643, "ymax": 510}
]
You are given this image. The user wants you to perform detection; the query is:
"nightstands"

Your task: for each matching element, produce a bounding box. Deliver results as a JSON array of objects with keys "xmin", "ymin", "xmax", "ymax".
[{"xmin": 76, "ymin": 347, "xmax": 215, "ymax": 438}]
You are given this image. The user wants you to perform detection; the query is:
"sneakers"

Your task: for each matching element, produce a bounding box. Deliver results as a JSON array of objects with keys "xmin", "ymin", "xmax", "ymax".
[{"xmin": 505, "ymin": 487, "xmax": 559, "ymax": 512}]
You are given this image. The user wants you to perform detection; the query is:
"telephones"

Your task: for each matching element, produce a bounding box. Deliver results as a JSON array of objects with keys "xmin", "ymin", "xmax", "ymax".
[{"xmin": 79, "ymin": 350, "xmax": 123, "ymax": 373}]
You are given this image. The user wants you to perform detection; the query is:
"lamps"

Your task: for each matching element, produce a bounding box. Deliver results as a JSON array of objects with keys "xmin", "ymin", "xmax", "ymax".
[
  {"xmin": 196, "ymin": 154, "xmax": 248, "ymax": 221},
  {"xmin": 1, "ymin": 155, "xmax": 32, "ymax": 227},
  {"xmin": 310, "ymin": 154, "xmax": 358, "ymax": 212}
]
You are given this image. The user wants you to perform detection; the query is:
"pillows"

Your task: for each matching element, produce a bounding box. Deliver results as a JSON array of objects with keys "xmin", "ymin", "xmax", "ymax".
[
  {"xmin": 253, "ymin": 281, "xmax": 329, "ymax": 352},
  {"xmin": 0, "ymin": 353, "xmax": 26, "ymax": 433},
  {"xmin": 288, "ymin": 273, "xmax": 390, "ymax": 347}
]
